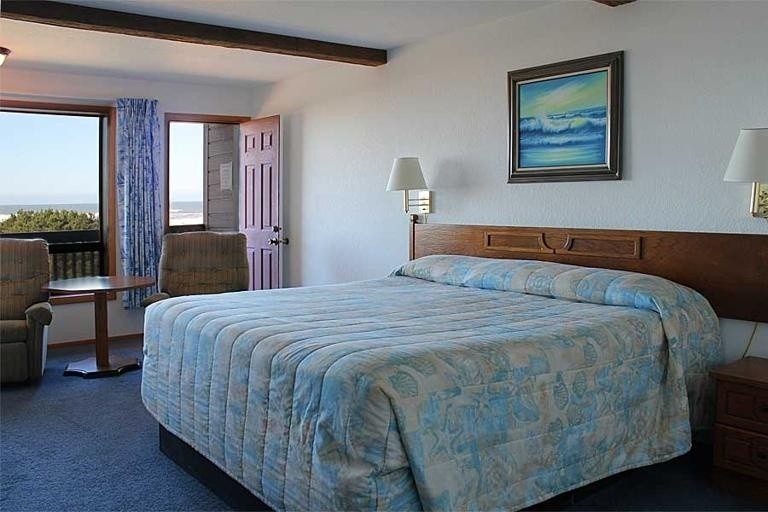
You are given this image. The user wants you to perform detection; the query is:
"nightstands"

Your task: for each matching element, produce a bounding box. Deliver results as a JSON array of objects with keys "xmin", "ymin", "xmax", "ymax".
[{"xmin": 708, "ymin": 357, "xmax": 768, "ymax": 480}]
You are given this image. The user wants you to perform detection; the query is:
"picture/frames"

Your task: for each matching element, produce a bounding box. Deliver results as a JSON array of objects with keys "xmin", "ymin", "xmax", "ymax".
[{"xmin": 507, "ymin": 51, "xmax": 623, "ymax": 183}]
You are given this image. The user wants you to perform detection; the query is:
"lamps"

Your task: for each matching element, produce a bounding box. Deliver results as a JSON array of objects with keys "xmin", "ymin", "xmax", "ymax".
[
  {"xmin": 385, "ymin": 157, "xmax": 432, "ymax": 214},
  {"xmin": 723, "ymin": 128, "xmax": 768, "ymax": 218}
]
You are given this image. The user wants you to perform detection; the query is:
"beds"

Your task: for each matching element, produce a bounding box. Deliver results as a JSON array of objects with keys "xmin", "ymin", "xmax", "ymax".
[{"xmin": 141, "ymin": 215, "xmax": 767, "ymax": 511}]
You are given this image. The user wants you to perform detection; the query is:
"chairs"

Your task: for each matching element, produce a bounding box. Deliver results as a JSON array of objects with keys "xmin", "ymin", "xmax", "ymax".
[
  {"xmin": 140, "ymin": 231, "xmax": 249, "ymax": 306},
  {"xmin": 0, "ymin": 238, "xmax": 53, "ymax": 383}
]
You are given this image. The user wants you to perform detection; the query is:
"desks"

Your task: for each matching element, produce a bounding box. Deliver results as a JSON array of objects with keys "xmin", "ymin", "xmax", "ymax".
[{"xmin": 41, "ymin": 276, "xmax": 155, "ymax": 379}]
